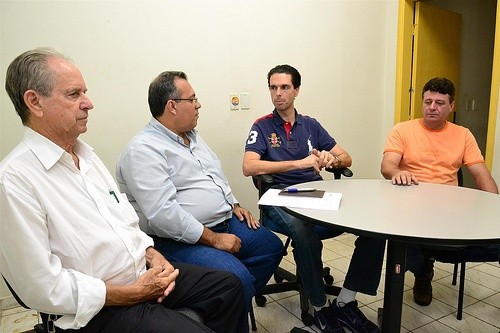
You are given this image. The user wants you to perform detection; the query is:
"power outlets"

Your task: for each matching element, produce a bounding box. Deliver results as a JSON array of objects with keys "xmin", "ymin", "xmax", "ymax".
[
  {"xmin": 467, "ymin": 98, "xmax": 473, "ymax": 113},
  {"xmin": 230, "ymin": 93, "xmax": 240, "ymax": 110}
]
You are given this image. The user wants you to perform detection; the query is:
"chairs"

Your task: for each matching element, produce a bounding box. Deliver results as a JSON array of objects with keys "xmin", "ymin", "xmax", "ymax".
[
  {"xmin": 0, "ymin": 274, "xmax": 205, "ymax": 333},
  {"xmin": 252, "ymin": 157, "xmax": 353, "ymax": 326},
  {"xmin": 438, "ymin": 165, "xmax": 475, "ymax": 319}
]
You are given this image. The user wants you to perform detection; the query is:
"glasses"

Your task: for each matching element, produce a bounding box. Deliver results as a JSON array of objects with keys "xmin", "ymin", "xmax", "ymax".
[{"xmin": 169, "ymin": 97, "xmax": 199, "ymax": 105}]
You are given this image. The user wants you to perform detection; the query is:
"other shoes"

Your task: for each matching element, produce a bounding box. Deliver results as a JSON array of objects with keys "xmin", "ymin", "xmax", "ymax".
[{"xmin": 413, "ymin": 265, "xmax": 434, "ymax": 306}]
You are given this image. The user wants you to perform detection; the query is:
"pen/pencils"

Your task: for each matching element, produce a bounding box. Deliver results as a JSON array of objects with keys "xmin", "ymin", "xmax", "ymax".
[
  {"xmin": 104, "ymin": 188, "xmax": 121, "ymax": 206},
  {"xmin": 280, "ymin": 187, "xmax": 318, "ymax": 194}
]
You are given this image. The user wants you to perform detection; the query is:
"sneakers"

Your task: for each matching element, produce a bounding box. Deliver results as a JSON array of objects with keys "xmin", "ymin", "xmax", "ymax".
[{"xmin": 312, "ymin": 298, "xmax": 379, "ymax": 333}]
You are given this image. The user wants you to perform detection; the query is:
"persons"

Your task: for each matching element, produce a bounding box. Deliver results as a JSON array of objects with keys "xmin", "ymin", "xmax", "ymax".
[
  {"xmin": 380, "ymin": 77, "xmax": 500, "ymax": 307},
  {"xmin": 117, "ymin": 71, "xmax": 284, "ymax": 314},
  {"xmin": 242, "ymin": 65, "xmax": 381, "ymax": 333},
  {"xmin": 0, "ymin": 47, "xmax": 249, "ymax": 333}
]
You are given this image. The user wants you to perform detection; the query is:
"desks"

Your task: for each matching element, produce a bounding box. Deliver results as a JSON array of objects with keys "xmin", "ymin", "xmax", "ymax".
[{"xmin": 282, "ymin": 180, "xmax": 500, "ymax": 333}]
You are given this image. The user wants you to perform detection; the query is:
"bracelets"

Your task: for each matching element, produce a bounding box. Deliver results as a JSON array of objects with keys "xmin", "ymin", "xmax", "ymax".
[{"xmin": 333, "ymin": 155, "xmax": 341, "ymax": 168}]
